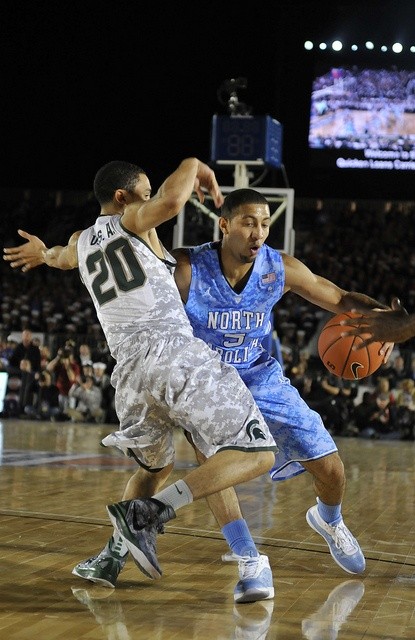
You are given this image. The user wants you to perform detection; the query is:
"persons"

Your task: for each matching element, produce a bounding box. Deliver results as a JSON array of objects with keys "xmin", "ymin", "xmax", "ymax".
[
  {"xmin": 339, "ymin": 295, "xmax": 415, "ymax": 354},
  {"xmin": 276, "ymin": 213, "xmax": 412, "ymax": 441},
  {"xmin": 307, "ymin": 66, "xmax": 415, "ymax": 150},
  {"xmin": 2, "ymin": 290, "xmax": 116, "ymax": 423},
  {"xmin": 3, "ymin": 156, "xmax": 277, "ymax": 594},
  {"xmin": 173, "ymin": 188, "xmax": 397, "ymax": 603}
]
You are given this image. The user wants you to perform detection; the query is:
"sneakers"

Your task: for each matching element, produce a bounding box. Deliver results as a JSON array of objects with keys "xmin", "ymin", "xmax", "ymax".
[
  {"xmin": 72, "ymin": 536, "xmax": 129, "ymax": 589},
  {"xmin": 221, "ymin": 552, "xmax": 275, "ymax": 602},
  {"xmin": 105, "ymin": 496, "xmax": 177, "ymax": 581},
  {"xmin": 306, "ymin": 503, "xmax": 366, "ymax": 576}
]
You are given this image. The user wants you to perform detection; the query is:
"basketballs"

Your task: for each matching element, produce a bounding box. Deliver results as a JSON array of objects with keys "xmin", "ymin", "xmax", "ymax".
[{"xmin": 317, "ymin": 313, "xmax": 385, "ymax": 381}]
